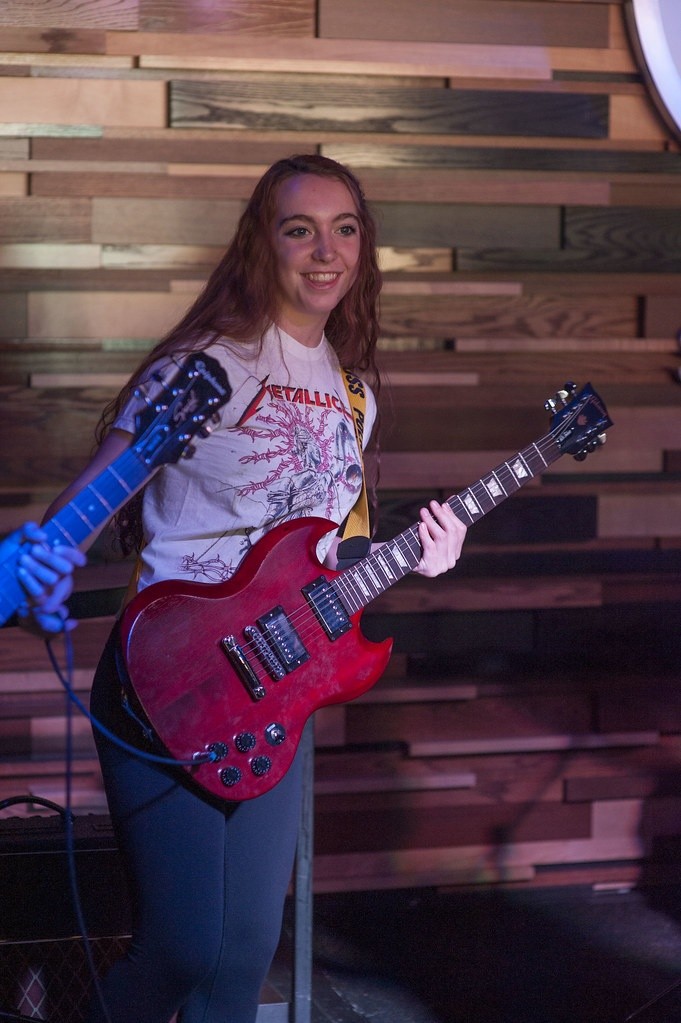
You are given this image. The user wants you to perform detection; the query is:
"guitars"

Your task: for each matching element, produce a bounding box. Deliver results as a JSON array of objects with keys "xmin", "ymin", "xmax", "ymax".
[
  {"xmin": 109, "ymin": 381, "xmax": 614, "ymax": 803},
  {"xmin": 0, "ymin": 348, "xmax": 232, "ymax": 634}
]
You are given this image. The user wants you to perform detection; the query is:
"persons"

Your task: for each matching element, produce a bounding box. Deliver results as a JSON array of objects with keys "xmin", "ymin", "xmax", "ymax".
[
  {"xmin": 35, "ymin": 156, "xmax": 467, "ymax": 1023},
  {"xmin": 0, "ymin": 522, "xmax": 88, "ymax": 630}
]
